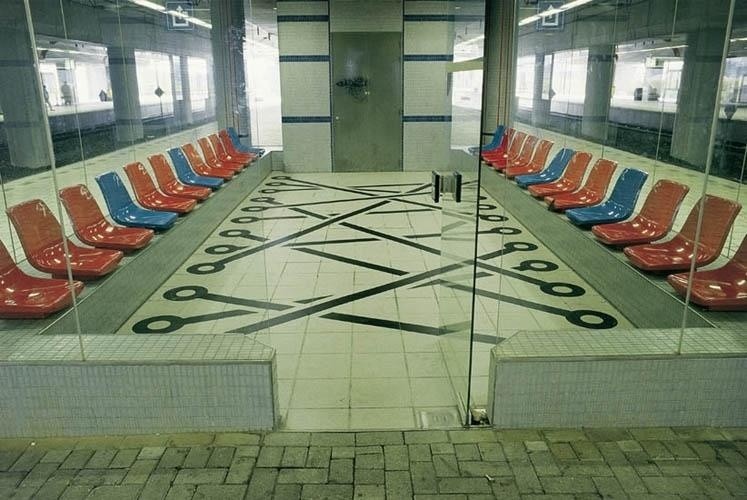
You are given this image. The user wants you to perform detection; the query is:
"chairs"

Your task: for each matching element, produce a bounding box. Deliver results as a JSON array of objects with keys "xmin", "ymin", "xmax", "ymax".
[
  {"xmin": 197, "ymin": 137, "xmax": 244, "ymax": 174},
  {"xmin": 528, "ymin": 152, "xmax": 593, "ymax": 198},
  {"xmin": 501, "ymin": 139, "xmax": 554, "ymax": 176},
  {"xmin": 513, "ymin": 147, "xmax": 576, "ymax": 185},
  {"xmin": 563, "ymin": 167, "xmax": 648, "ymax": 229},
  {"xmin": 218, "ymin": 130, "xmax": 258, "ymax": 162},
  {"xmin": 666, "ymin": 235, "xmax": 746, "ymax": 313},
  {"xmin": 476, "ymin": 128, "xmax": 514, "ymax": 160},
  {"xmin": 544, "ymin": 158, "xmax": 618, "ymax": 209},
  {"xmin": 180, "ymin": 143, "xmax": 235, "ymax": 181},
  {"xmin": 122, "ymin": 160, "xmax": 198, "ymax": 214},
  {"xmin": 226, "ymin": 126, "xmax": 264, "ymax": 155},
  {"xmin": 166, "ymin": 147, "xmax": 225, "ymax": 189},
  {"xmin": 57, "ymin": 183, "xmax": 155, "ymax": 251},
  {"xmin": 482, "ymin": 131, "xmax": 528, "ymax": 165},
  {"xmin": 207, "ymin": 133, "xmax": 251, "ymax": 168},
  {"xmin": 4, "ymin": 198, "xmax": 124, "ymax": 279},
  {"xmin": 0, "ymin": 239, "xmax": 83, "ymax": 318},
  {"xmin": 622, "ymin": 193, "xmax": 741, "ymax": 271},
  {"xmin": 93, "ymin": 170, "xmax": 177, "ymax": 231},
  {"xmin": 467, "ymin": 125, "xmax": 505, "ymax": 155},
  {"xmin": 492, "ymin": 135, "xmax": 539, "ymax": 171},
  {"xmin": 591, "ymin": 179, "xmax": 690, "ymax": 247},
  {"xmin": 146, "ymin": 152, "xmax": 212, "ymax": 202}
]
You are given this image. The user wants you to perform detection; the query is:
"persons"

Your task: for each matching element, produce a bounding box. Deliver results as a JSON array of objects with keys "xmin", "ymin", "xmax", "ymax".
[
  {"xmin": 60, "ymin": 80, "xmax": 74, "ymax": 106},
  {"xmin": 42, "ymin": 84, "xmax": 55, "ymax": 111},
  {"xmin": 99, "ymin": 89, "xmax": 108, "ymax": 102},
  {"xmin": 722, "ymin": 97, "xmax": 738, "ymax": 119},
  {"xmin": 648, "ymin": 88, "xmax": 660, "ymax": 101}
]
